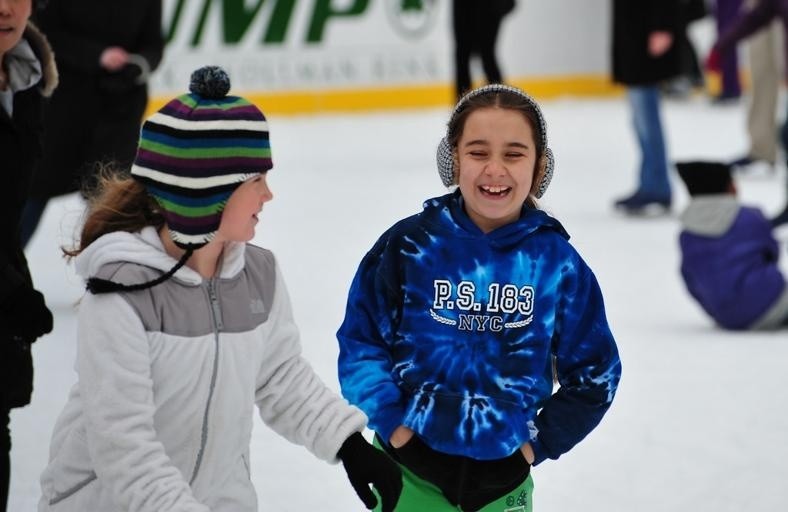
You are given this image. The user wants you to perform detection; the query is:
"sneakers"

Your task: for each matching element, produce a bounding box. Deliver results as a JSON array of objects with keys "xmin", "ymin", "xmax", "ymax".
[{"xmin": 617, "ymin": 192, "xmax": 671, "ymax": 209}]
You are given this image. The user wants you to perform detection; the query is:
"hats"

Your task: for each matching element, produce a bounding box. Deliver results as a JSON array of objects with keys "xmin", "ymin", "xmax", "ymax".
[
  {"xmin": 130, "ymin": 66, "xmax": 272, "ymax": 253},
  {"xmin": 674, "ymin": 162, "xmax": 727, "ymax": 194}
]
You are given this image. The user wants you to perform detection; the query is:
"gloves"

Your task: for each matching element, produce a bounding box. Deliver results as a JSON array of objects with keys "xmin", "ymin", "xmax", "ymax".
[
  {"xmin": 395, "ymin": 433, "xmax": 531, "ymax": 511},
  {"xmin": 338, "ymin": 434, "xmax": 402, "ymax": 511}
]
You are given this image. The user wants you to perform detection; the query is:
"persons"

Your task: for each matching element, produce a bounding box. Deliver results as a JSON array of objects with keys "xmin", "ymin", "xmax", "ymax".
[
  {"xmin": 36, "ymin": 64, "xmax": 404, "ymax": 512},
  {"xmin": 669, "ymin": 159, "xmax": 787, "ymax": 330},
  {"xmin": 704, "ymin": 0, "xmax": 788, "ymax": 170},
  {"xmin": 450, "ymin": 0, "xmax": 517, "ymax": 105},
  {"xmin": 29, "ymin": 0, "xmax": 164, "ymax": 186},
  {"xmin": 606, "ymin": 1, "xmax": 713, "ymax": 213},
  {"xmin": 0, "ymin": 1, "xmax": 63, "ymax": 511},
  {"xmin": 333, "ymin": 79, "xmax": 625, "ymax": 511}
]
